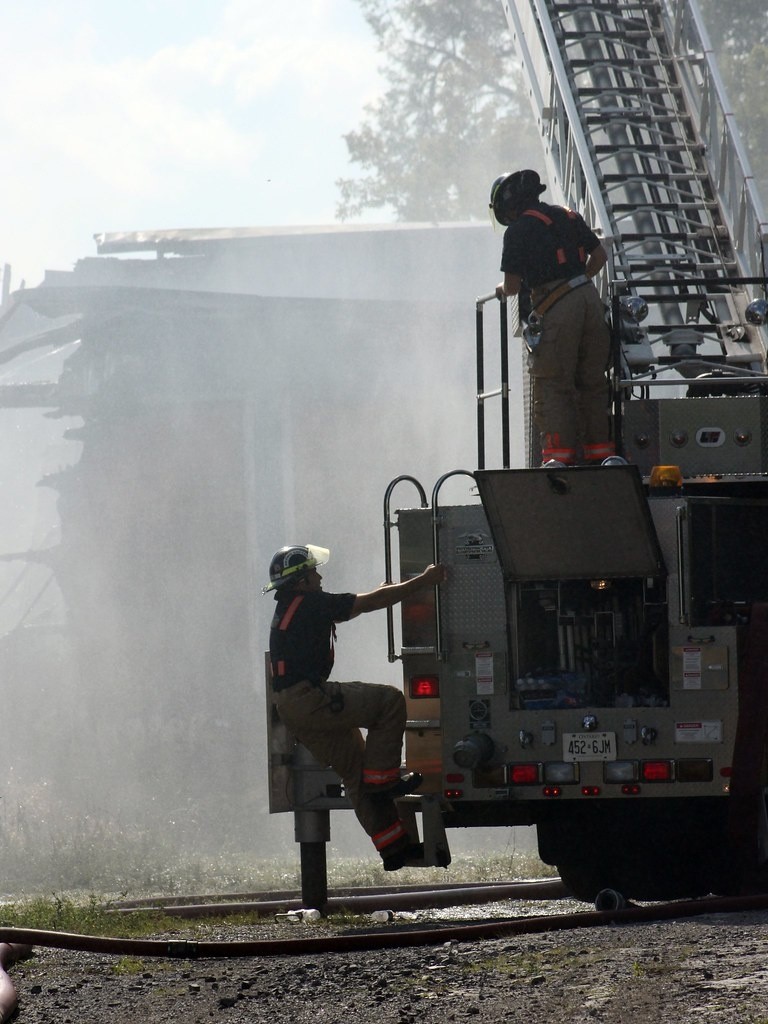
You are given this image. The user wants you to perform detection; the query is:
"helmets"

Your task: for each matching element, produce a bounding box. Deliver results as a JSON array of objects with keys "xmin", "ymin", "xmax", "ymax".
[
  {"xmin": 489, "ymin": 169, "xmax": 547, "ymax": 226},
  {"xmin": 261, "ymin": 545, "xmax": 318, "ymax": 601}
]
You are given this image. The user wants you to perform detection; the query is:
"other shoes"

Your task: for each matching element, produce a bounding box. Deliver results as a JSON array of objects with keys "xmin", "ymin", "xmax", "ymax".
[
  {"xmin": 383, "ymin": 843, "xmax": 424, "ymax": 871},
  {"xmin": 401, "ymin": 772, "xmax": 423, "ymax": 796}
]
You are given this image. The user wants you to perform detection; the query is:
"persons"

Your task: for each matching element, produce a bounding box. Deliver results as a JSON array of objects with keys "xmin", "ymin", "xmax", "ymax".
[
  {"xmin": 268, "ymin": 543, "xmax": 447, "ymax": 873},
  {"xmin": 487, "ymin": 167, "xmax": 614, "ymax": 468}
]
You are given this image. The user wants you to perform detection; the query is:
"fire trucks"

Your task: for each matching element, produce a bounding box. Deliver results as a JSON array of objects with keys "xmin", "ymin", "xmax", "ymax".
[{"xmin": 263, "ymin": 0, "xmax": 767, "ymax": 907}]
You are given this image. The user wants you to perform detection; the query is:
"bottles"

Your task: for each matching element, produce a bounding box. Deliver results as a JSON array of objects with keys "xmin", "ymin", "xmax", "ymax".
[
  {"xmin": 527, "ymin": 679, "xmax": 546, "ymax": 709},
  {"xmin": 538, "ymin": 680, "xmax": 560, "ymax": 710},
  {"xmin": 287, "ymin": 908, "xmax": 320, "ymax": 922},
  {"xmin": 372, "ymin": 910, "xmax": 393, "ymax": 923},
  {"xmin": 516, "ymin": 679, "xmax": 536, "ymax": 710}
]
